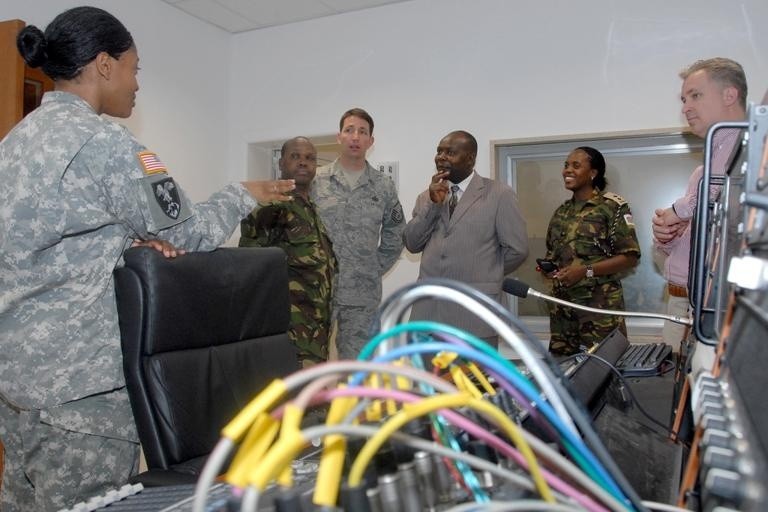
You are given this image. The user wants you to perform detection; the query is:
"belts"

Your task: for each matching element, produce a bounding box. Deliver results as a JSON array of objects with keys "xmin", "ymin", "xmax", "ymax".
[{"xmin": 668, "ymin": 282, "xmax": 687, "ymax": 298}]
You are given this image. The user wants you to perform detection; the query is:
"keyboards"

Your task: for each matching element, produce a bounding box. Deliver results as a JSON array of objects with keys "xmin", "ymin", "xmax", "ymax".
[{"xmin": 613, "ymin": 342, "xmax": 672, "ymax": 371}]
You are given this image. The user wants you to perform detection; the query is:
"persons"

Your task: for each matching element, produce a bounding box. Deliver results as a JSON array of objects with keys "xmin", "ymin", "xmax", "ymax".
[
  {"xmin": 403, "ymin": 131, "xmax": 527, "ymax": 379},
  {"xmin": 536, "ymin": 146, "xmax": 641, "ymax": 358},
  {"xmin": 651, "ymin": 56, "xmax": 748, "ymax": 371},
  {"xmin": 1, "ymin": 5, "xmax": 297, "ymax": 512},
  {"xmin": 306, "ymin": 109, "xmax": 407, "ymax": 382},
  {"xmin": 240, "ymin": 136, "xmax": 338, "ymax": 392}
]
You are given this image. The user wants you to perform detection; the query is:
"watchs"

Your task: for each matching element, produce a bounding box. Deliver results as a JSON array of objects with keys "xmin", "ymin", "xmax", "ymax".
[{"xmin": 585, "ymin": 263, "xmax": 594, "ymax": 278}]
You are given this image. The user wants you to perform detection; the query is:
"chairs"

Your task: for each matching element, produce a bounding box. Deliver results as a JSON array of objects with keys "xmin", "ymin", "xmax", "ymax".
[{"xmin": 113, "ymin": 245, "xmax": 302, "ymax": 472}]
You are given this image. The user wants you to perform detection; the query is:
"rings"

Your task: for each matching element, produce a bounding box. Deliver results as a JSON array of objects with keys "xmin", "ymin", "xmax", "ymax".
[{"xmin": 274, "ymin": 185, "xmax": 280, "ymax": 193}]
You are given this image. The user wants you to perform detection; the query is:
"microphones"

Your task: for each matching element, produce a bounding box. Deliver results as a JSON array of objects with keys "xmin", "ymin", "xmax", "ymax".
[{"xmin": 502, "ymin": 277, "xmax": 695, "ymax": 327}]
